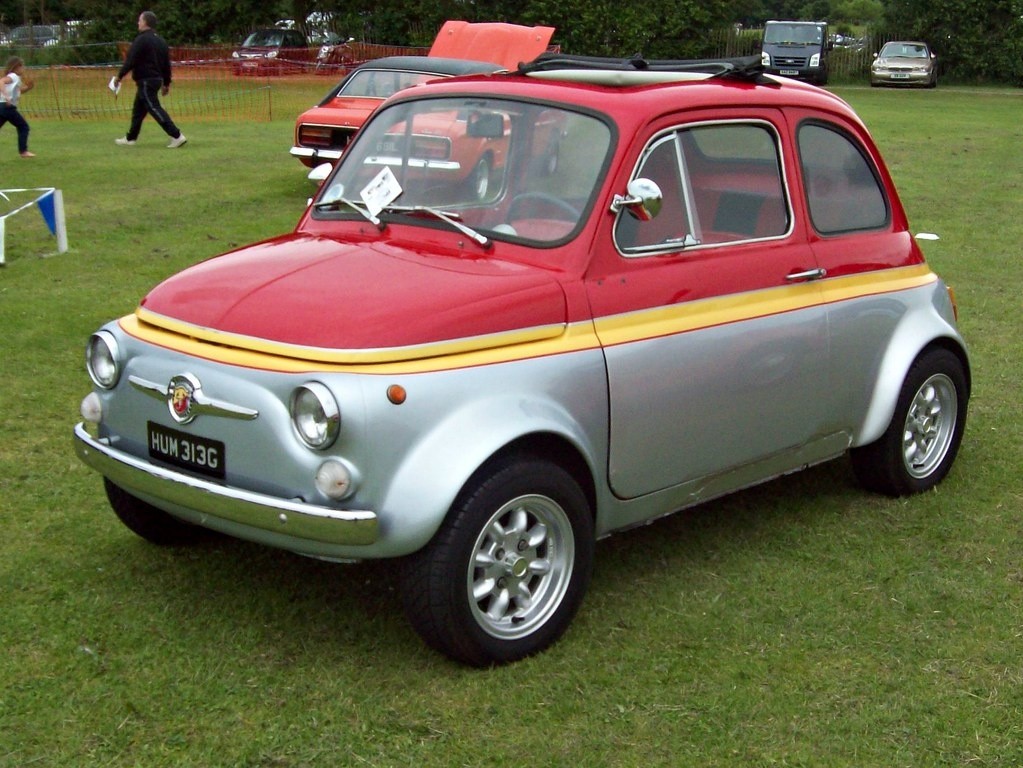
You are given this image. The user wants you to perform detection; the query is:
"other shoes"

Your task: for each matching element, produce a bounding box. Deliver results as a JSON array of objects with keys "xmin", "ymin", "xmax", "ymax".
[{"xmin": 19, "ymin": 152, "xmax": 36, "ymax": 158}]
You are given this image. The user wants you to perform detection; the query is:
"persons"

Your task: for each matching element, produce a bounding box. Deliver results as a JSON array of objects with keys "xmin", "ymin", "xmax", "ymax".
[
  {"xmin": 114, "ymin": 11, "xmax": 187, "ymax": 147},
  {"xmin": 0, "ymin": 56, "xmax": 35, "ymax": 157}
]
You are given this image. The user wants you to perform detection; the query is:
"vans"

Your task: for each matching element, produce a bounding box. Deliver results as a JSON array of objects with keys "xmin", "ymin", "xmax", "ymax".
[{"xmin": 761, "ymin": 20, "xmax": 829, "ymax": 85}]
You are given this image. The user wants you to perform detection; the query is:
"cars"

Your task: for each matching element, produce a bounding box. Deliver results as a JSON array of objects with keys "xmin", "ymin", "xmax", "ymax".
[
  {"xmin": 230, "ymin": 27, "xmax": 310, "ymax": 75},
  {"xmin": 290, "ymin": 17, "xmax": 570, "ymax": 201},
  {"xmin": 869, "ymin": 39, "xmax": 938, "ymax": 89},
  {"xmin": 72, "ymin": 53, "xmax": 975, "ymax": 670}
]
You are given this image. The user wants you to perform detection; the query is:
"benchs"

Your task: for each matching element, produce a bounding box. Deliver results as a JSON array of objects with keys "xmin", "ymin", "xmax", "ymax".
[{"xmin": 639, "ymin": 163, "xmax": 847, "ymax": 245}]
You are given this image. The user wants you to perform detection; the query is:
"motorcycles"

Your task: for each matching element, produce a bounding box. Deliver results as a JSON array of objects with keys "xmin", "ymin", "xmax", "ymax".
[{"xmin": 315, "ymin": 30, "xmax": 355, "ymax": 73}]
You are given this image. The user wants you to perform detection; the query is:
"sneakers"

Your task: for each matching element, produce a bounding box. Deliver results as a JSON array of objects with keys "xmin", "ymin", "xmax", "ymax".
[
  {"xmin": 115, "ymin": 135, "xmax": 136, "ymax": 146},
  {"xmin": 168, "ymin": 133, "xmax": 186, "ymax": 148}
]
